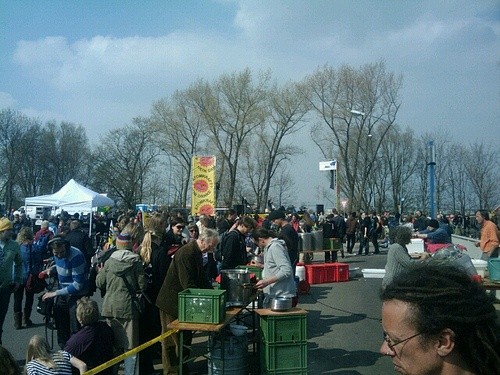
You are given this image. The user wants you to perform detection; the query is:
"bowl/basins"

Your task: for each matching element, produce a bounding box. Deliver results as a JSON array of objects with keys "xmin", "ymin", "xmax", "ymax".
[
  {"xmin": 269, "ymin": 296, "xmax": 292, "ymax": 311},
  {"xmin": 349, "ymin": 268, "xmax": 356, "ymax": 278}
]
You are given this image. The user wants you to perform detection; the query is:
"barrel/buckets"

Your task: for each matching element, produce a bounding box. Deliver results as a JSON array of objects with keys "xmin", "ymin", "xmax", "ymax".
[
  {"xmin": 487, "ymin": 258, "xmax": 500, "ymax": 282},
  {"xmin": 220, "ymin": 269, "xmax": 251, "ymax": 307},
  {"xmin": 208, "ymin": 324, "xmax": 248, "ymax": 375},
  {"xmin": 298, "ymin": 232, "xmax": 311, "ymax": 251},
  {"xmin": 310, "ymin": 230, "xmax": 323, "ymax": 251}
]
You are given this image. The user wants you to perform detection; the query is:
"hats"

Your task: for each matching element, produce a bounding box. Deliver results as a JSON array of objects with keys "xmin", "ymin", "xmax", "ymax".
[
  {"xmin": 116, "ymin": 233, "xmax": 133, "ymax": 250},
  {"xmin": 428, "ymin": 219, "xmax": 440, "ymax": 228},
  {"xmin": 0, "ymin": 217, "xmax": 13, "ymax": 232},
  {"xmin": 269, "ymin": 210, "xmax": 285, "ymax": 221}
]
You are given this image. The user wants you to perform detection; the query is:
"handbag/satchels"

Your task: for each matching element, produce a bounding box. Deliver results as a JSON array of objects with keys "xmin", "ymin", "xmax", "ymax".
[
  {"xmin": 37, "ymin": 293, "xmax": 54, "ymax": 314},
  {"xmin": 132, "ymin": 289, "xmax": 154, "ymax": 316},
  {"xmin": 374, "ymin": 223, "xmax": 382, "ymax": 233},
  {"xmin": 26, "ymin": 274, "xmax": 45, "ymax": 294}
]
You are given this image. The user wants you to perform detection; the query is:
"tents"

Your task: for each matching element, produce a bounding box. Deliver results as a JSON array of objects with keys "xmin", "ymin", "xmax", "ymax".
[{"xmin": 25, "ymin": 179, "xmax": 115, "ymax": 236}]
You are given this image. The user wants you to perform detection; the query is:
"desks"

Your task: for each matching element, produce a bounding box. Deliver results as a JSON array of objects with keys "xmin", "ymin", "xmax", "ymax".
[
  {"xmin": 167, "ymin": 291, "xmax": 259, "ymax": 375},
  {"xmin": 299, "ymin": 248, "xmax": 343, "ymax": 265},
  {"xmin": 482, "ymin": 279, "xmax": 500, "ymax": 290}
]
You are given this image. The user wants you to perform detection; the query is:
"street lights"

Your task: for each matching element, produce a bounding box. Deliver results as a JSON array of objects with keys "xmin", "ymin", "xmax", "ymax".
[{"xmin": 351, "ymin": 109, "xmax": 405, "ymax": 213}]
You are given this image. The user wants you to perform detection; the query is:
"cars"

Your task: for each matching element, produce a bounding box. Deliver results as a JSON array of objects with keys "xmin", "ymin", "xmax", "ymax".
[{"xmin": 13, "ymin": 206, "xmax": 25, "ymax": 215}]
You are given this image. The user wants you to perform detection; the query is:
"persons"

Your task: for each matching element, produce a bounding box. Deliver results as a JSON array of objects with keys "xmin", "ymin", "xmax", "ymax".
[
  {"xmin": 395, "ymin": 211, "xmax": 498, "ymax": 244},
  {"xmin": 288, "ymin": 209, "xmax": 401, "ymax": 263},
  {"xmin": 475, "ymin": 210, "xmax": 500, "ymax": 276},
  {"xmin": 380, "ymin": 266, "xmax": 500, "ymax": 375},
  {"xmin": 0, "ymin": 208, "xmax": 237, "ymax": 375},
  {"xmin": 216, "ymin": 210, "xmax": 299, "ymax": 309},
  {"xmin": 383, "ymin": 226, "xmax": 428, "ymax": 288}
]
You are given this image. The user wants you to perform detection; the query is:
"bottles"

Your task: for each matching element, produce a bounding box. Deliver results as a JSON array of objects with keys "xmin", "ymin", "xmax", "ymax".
[{"xmin": 295, "ymin": 262, "xmax": 305, "ymax": 282}]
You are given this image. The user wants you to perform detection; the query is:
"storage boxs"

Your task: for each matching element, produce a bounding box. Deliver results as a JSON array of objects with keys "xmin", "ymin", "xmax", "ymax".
[
  {"xmin": 236, "ymin": 265, "xmax": 263, "ymax": 282},
  {"xmin": 322, "ymin": 238, "xmax": 342, "ymax": 249},
  {"xmin": 471, "ymin": 259, "xmax": 487, "ymax": 278},
  {"xmin": 405, "ymin": 238, "xmax": 424, "ymax": 254},
  {"xmin": 362, "ymin": 269, "xmax": 385, "ymax": 279},
  {"xmin": 297, "ymin": 262, "xmax": 349, "ymax": 285},
  {"xmin": 177, "ymin": 288, "xmax": 227, "ymax": 325},
  {"xmin": 254, "ymin": 309, "xmax": 307, "ymax": 375}
]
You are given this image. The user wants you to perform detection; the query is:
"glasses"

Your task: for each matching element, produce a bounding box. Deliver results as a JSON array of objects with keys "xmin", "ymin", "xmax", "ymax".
[
  {"xmin": 190, "ymin": 229, "xmax": 197, "ymax": 232},
  {"xmin": 383, "ymin": 332, "xmax": 420, "ymax": 351},
  {"xmin": 176, "ymin": 226, "xmax": 185, "ymax": 229}
]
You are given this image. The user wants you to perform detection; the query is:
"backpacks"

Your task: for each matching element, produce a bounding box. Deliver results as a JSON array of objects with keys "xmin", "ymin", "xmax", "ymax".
[
  {"xmin": 215, "ymin": 230, "xmax": 240, "ymax": 262},
  {"xmin": 36, "ymin": 231, "xmax": 51, "ymax": 249}
]
